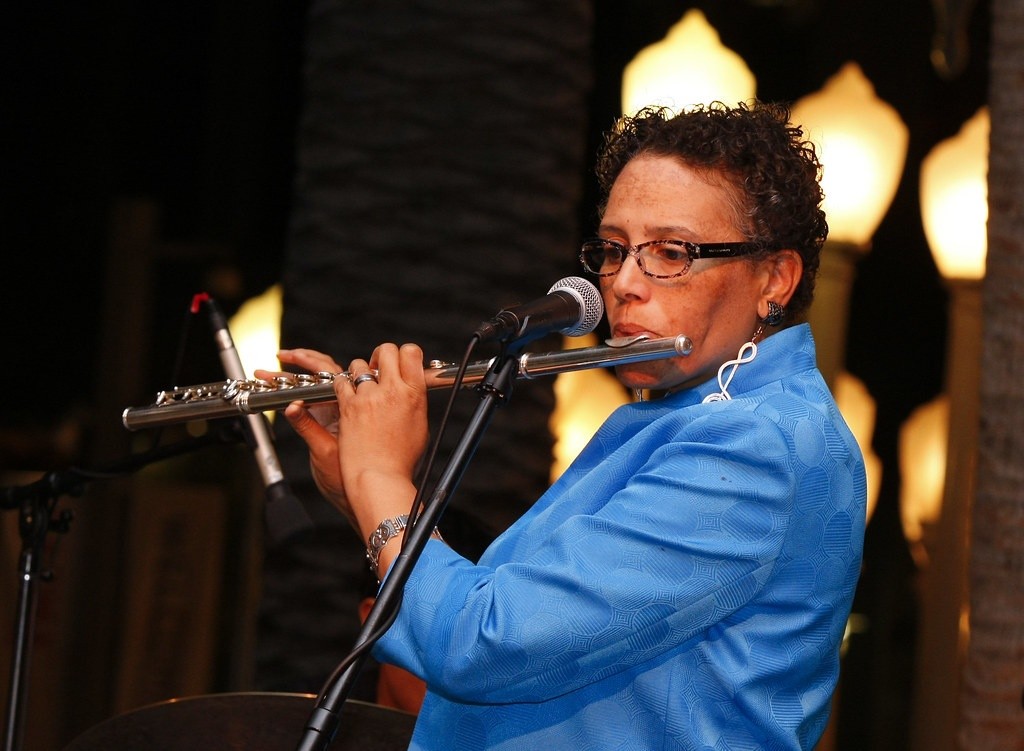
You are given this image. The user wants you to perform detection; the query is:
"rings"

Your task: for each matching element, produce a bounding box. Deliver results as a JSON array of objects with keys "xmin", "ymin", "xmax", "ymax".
[{"xmin": 354, "ymin": 374, "xmax": 376, "ymax": 387}]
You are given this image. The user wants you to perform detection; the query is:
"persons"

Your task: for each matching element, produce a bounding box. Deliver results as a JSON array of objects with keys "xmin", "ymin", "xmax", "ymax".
[{"xmin": 253, "ymin": 100, "xmax": 867, "ymax": 751}]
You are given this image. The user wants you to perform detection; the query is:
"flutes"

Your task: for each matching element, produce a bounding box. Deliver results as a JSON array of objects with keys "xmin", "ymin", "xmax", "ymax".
[{"xmin": 118, "ymin": 336, "xmax": 693, "ymax": 432}]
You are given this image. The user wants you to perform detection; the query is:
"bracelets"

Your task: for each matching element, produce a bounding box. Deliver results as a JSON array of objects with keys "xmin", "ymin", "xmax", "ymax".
[{"xmin": 365, "ymin": 514, "xmax": 444, "ymax": 574}]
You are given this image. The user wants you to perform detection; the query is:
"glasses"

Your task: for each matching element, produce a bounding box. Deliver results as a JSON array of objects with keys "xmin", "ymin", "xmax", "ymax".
[{"xmin": 580, "ymin": 239, "xmax": 783, "ymax": 279}]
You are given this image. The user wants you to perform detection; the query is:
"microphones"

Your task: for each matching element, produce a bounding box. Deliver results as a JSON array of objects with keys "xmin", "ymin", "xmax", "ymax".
[
  {"xmin": 475, "ymin": 277, "xmax": 604, "ymax": 343},
  {"xmin": 202, "ymin": 298, "xmax": 284, "ymax": 487}
]
